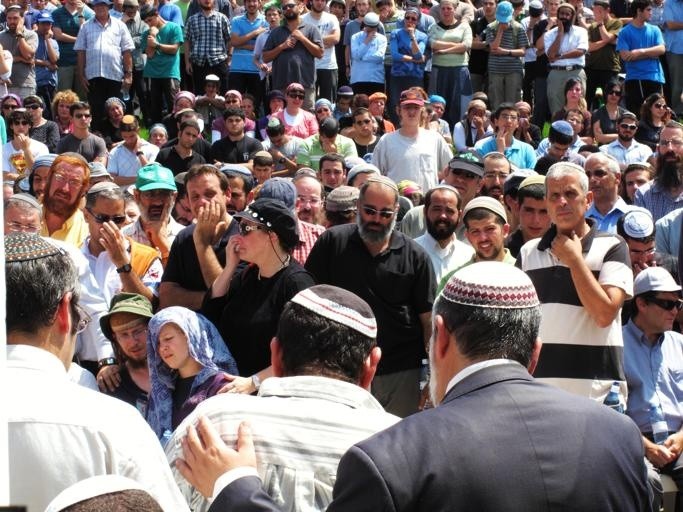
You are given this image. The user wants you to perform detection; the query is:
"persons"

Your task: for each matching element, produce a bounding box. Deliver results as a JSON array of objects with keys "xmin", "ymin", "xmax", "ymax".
[{"xmin": 0, "ymin": 2, "xmax": 682, "ymax": 512}]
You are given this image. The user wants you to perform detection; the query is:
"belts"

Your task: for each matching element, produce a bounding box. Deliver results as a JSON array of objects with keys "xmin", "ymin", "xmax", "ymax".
[{"xmin": 550, "ymin": 65, "xmax": 584, "ymax": 71}]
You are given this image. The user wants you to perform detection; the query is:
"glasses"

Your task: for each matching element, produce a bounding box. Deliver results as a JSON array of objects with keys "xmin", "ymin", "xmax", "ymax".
[
  {"xmin": 57, "ymin": 290, "xmax": 92, "ymax": 334},
  {"xmin": 289, "ymin": 94, "xmax": 304, "ymax": 99},
  {"xmin": 59, "ymin": 105, "xmax": 70, "ymax": 108},
  {"xmin": 608, "ymin": 90, "xmax": 621, "ymax": 96},
  {"xmin": 316, "ymin": 108, "xmax": 328, "ymax": 113},
  {"xmin": 85, "ymin": 207, "xmax": 125, "ymax": 224},
  {"xmin": 630, "ymin": 247, "xmax": 657, "ymax": 257},
  {"xmin": 4, "ymin": 222, "xmax": 41, "ymax": 232},
  {"xmin": 26, "ymin": 105, "xmax": 41, "ymax": 110},
  {"xmin": 185, "ymin": 131, "xmax": 197, "ymax": 138},
  {"xmin": 14, "ymin": 121, "xmax": 29, "ymax": 125},
  {"xmin": 112, "ymin": 327, "xmax": 148, "ymax": 338},
  {"xmin": 54, "ymin": 174, "xmax": 84, "ymax": 189},
  {"xmin": 649, "ymin": 299, "xmax": 683, "ymax": 310},
  {"xmin": 485, "ymin": 173, "xmax": 509, "ymax": 180},
  {"xmin": 178, "ymin": 200, "xmax": 193, "ymax": 213},
  {"xmin": 620, "ymin": 123, "xmax": 637, "ymax": 130},
  {"xmin": 2, "ymin": 104, "xmax": 19, "ymax": 110},
  {"xmin": 298, "ymin": 197, "xmax": 321, "ymax": 205},
  {"xmin": 361, "ymin": 207, "xmax": 395, "ymax": 218},
  {"xmin": 405, "ymin": 16, "xmax": 417, "ymax": 21},
  {"xmin": 501, "ymin": 115, "xmax": 517, "ymax": 119},
  {"xmin": 75, "ymin": 114, "xmax": 90, "ymax": 118},
  {"xmin": 587, "ymin": 169, "xmax": 615, "ymax": 178},
  {"xmin": 282, "ymin": 4, "xmax": 296, "ymax": 9},
  {"xmin": 226, "ymin": 100, "xmax": 238, "ymax": 104},
  {"xmin": 654, "ymin": 103, "xmax": 667, "ymax": 109},
  {"xmin": 237, "ymin": 222, "xmax": 270, "ymax": 236},
  {"xmin": 357, "ymin": 120, "xmax": 371, "ymax": 125}
]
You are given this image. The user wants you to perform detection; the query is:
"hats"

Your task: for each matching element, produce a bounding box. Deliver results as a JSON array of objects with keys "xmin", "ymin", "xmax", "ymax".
[
  {"xmin": 233, "ymin": 197, "xmax": 298, "ymax": 236},
  {"xmin": 87, "ymin": 182, "xmax": 119, "ymax": 193},
  {"xmin": 624, "ymin": 212, "xmax": 653, "ymax": 238},
  {"xmin": 89, "ymin": 162, "xmax": 114, "ymax": 181},
  {"xmin": 32, "ymin": 153, "xmax": 59, "ymax": 167},
  {"xmin": 268, "ymin": 117, "xmax": 282, "ymax": 127},
  {"xmin": 496, "ymin": 1, "xmax": 514, "ymax": 23},
  {"xmin": 462, "ymin": 196, "xmax": 507, "ymax": 223},
  {"xmin": 136, "ymin": 162, "xmax": 177, "ymax": 191},
  {"xmin": 441, "ymin": 261, "xmax": 539, "ymax": 309},
  {"xmin": 119, "ymin": 115, "xmax": 138, "ymax": 130},
  {"xmin": 634, "ymin": 267, "xmax": 682, "ymax": 295},
  {"xmin": 269, "ymin": 90, "xmax": 288, "ymax": 100},
  {"xmin": 220, "ymin": 163, "xmax": 252, "ymax": 176},
  {"xmin": 397, "ymin": 179, "xmax": 422, "ymax": 196},
  {"xmin": 363, "ymin": 12, "xmax": 379, "ymax": 26},
  {"xmin": 123, "ymin": 0, "xmax": 139, "ymax": 6},
  {"xmin": 11, "ymin": 192, "xmax": 43, "ymax": 215},
  {"xmin": 400, "ymin": 90, "xmax": 424, "ymax": 105},
  {"xmin": 347, "ymin": 164, "xmax": 380, "ymax": 184},
  {"xmin": 552, "ymin": 120, "xmax": 574, "ymax": 135},
  {"xmin": 325, "ymin": 185, "xmax": 360, "ymax": 211},
  {"xmin": 291, "ymin": 283, "xmax": 378, "ymax": 338},
  {"xmin": 255, "ymin": 178, "xmax": 297, "ymax": 210},
  {"xmin": 338, "ymin": 86, "xmax": 354, "ymax": 96},
  {"xmin": 207, "ymin": 74, "xmax": 220, "ymax": 80},
  {"xmin": 100, "ymin": 292, "xmax": 154, "ymax": 341},
  {"xmin": 529, "ymin": 0, "xmax": 543, "ymax": 17},
  {"xmin": 5, "ymin": 233, "xmax": 59, "ymax": 262},
  {"xmin": 518, "ymin": 175, "xmax": 546, "ymax": 190},
  {"xmin": 255, "ymin": 151, "xmax": 272, "ymax": 158},
  {"xmin": 429, "ymin": 94, "xmax": 447, "ymax": 105},
  {"xmin": 450, "ymin": 150, "xmax": 484, "ymax": 178},
  {"xmin": 36, "ymin": 10, "xmax": 54, "ymax": 22},
  {"xmin": 369, "ymin": 92, "xmax": 387, "ymax": 100},
  {"xmin": 87, "ymin": 0, "xmax": 114, "ymax": 8},
  {"xmin": 504, "ymin": 169, "xmax": 539, "ymax": 194},
  {"xmin": 368, "ymin": 176, "xmax": 398, "ymax": 193}
]
